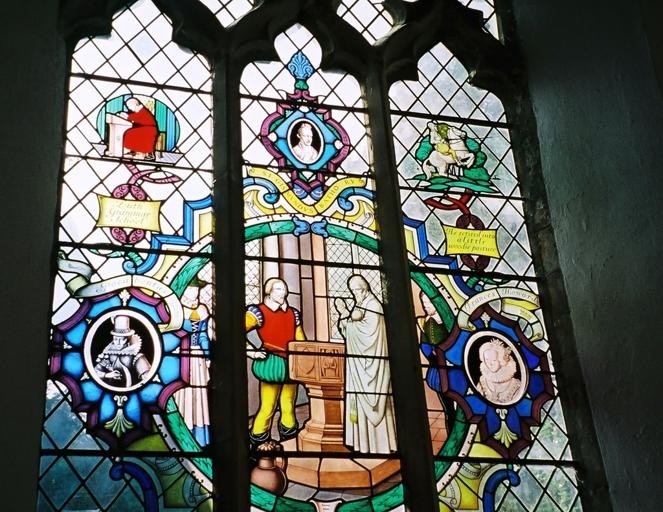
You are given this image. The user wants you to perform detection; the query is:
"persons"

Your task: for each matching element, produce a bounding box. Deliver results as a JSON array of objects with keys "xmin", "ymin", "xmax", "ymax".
[
  {"xmin": 477, "ymin": 337, "xmax": 521, "ymax": 403},
  {"xmin": 427, "ymin": 122, "xmax": 462, "ymax": 166},
  {"xmin": 334, "ymin": 274, "xmax": 398, "ymax": 454},
  {"xmin": 94, "ymin": 316, "xmax": 152, "ymax": 387},
  {"xmin": 293, "ymin": 123, "xmax": 318, "ymax": 162},
  {"xmin": 116, "ymin": 97, "xmax": 159, "ymax": 161},
  {"xmin": 245, "ymin": 277, "xmax": 309, "ymax": 458}
]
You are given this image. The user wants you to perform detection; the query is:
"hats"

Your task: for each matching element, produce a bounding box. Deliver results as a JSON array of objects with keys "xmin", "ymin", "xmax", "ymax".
[{"xmin": 110, "ymin": 315, "xmax": 135, "ymax": 337}]
